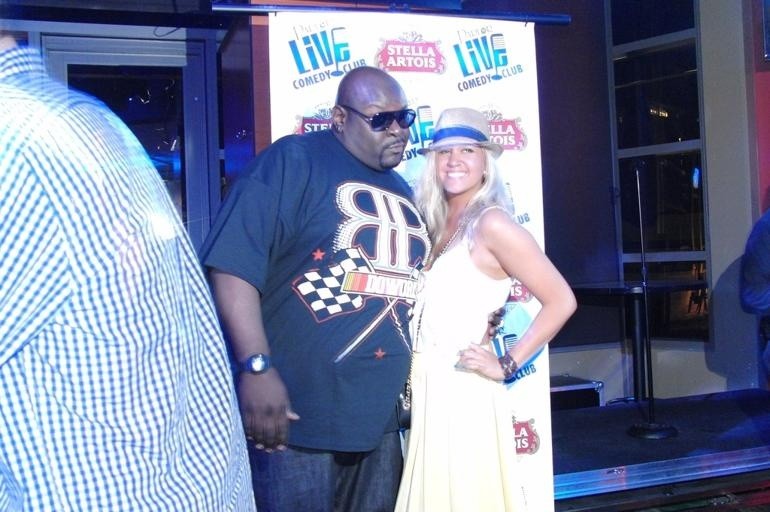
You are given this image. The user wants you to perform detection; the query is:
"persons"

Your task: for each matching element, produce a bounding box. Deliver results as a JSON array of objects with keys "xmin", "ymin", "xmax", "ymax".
[
  {"xmin": 1, "ymin": 20, "xmax": 258, "ymax": 512},
  {"xmin": 392, "ymin": 104, "xmax": 580, "ymax": 512},
  {"xmin": 198, "ymin": 63, "xmax": 510, "ymax": 512},
  {"xmin": 739, "ymin": 207, "xmax": 769, "ymax": 387}
]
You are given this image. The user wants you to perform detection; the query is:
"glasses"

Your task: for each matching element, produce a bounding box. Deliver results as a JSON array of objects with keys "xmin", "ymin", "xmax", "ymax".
[{"xmin": 337, "ymin": 103, "xmax": 417, "ymax": 133}]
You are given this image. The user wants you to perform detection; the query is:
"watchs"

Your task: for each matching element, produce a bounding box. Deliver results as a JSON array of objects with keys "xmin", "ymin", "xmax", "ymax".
[{"xmin": 237, "ymin": 353, "xmax": 278, "ymax": 375}]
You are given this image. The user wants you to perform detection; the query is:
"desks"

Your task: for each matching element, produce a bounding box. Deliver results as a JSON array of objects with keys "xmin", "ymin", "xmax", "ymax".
[{"xmin": 569, "ymin": 279, "xmax": 708, "ymax": 407}]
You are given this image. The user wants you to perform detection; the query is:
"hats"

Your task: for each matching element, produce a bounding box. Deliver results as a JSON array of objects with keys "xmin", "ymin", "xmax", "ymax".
[{"xmin": 426, "ymin": 108, "xmax": 504, "ymax": 161}]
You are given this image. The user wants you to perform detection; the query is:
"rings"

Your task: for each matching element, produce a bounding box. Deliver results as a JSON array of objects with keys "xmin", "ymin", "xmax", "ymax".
[{"xmin": 498, "ymin": 320, "xmax": 505, "ymax": 331}]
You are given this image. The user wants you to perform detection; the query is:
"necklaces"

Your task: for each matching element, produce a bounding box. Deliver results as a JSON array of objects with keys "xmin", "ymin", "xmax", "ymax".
[{"xmin": 424, "ymin": 214, "xmax": 471, "ymax": 268}]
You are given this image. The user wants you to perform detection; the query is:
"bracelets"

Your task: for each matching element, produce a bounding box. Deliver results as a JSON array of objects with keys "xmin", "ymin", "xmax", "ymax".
[{"xmin": 498, "ymin": 351, "xmax": 518, "ymax": 379}]
